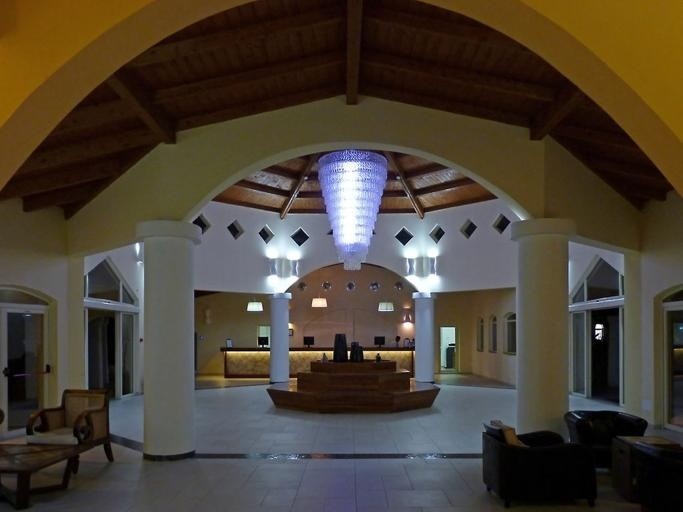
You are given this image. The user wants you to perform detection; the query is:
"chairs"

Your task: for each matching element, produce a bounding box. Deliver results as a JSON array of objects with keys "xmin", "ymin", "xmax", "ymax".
[
  {"xmin": 25, "ymin": 387, "xmax": 114, "ymax": 474},
  {"xmin": 483, "ymin": 430, "xmax": 598, "ymax": 511},
  {"xmin": 564, "ymin": 410, "xmax": 648, "ymax": 470}
]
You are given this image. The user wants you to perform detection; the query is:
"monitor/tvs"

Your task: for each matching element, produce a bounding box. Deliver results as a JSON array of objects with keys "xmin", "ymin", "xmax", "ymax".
[
  {"xmin": 258, "ymin": 336, "xmax": 268, "ymax": 348},
  {"xmin": 303, "ymin": 336, "xmax": 314, "ymax": 348},
  {"xmin": 374, "ymin": 336, "xmax": 385, "ymax": 347}
]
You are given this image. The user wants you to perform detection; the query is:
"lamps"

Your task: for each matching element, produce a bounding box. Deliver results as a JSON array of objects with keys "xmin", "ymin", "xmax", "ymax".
[
  {"xmin": 401, "ymin": 308, "xmax": 414, "ymax": 325},
  {"xmin": 312, "ymin": 293, "xmax": 327, "ymax": 308},
  {"xmin": 246, "ymin": 295, "xmax": 264, "ymax": 312},
  {"xmin": 289, "ymin": 308, "xmax": 293, "ymax": 324},
  {"xmin": 378, "ymin": 299, "xmax": 395, "ymax": 312},
  {"xmin": 317, "ymin": 150, "xmax": 389, "ymax": 271},
  {"xmin": 429, "ymin": 257, "xmax": 436, "ymax": 274},
  {"xmin": 290, "ymin": 260, "xmax": 299, "ymax": 276},
  {"xmin": 408, "ymin": 257, "xmax": 415, "ymax": 273},
  {"xmin": 270, "ymin": 259, "xmax": 277, "ymax": 274}
]
